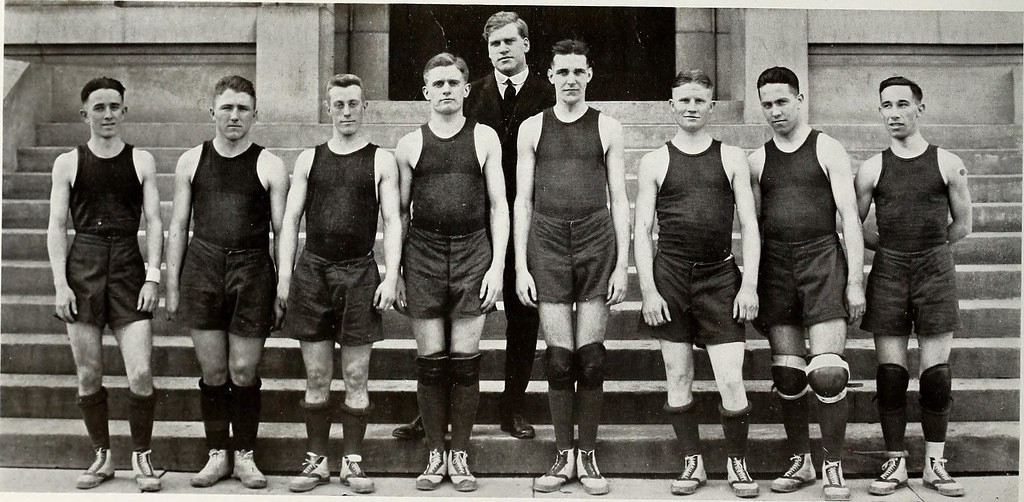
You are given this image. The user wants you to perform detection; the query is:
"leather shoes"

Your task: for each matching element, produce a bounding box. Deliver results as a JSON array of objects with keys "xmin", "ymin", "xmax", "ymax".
[
  {"xmin": 391, "ymin": 412, "xmax": 424, "ymax": 438},
  {"xmin": 500, "ymin": 408, "xmax": 535, "ymax": 438}
]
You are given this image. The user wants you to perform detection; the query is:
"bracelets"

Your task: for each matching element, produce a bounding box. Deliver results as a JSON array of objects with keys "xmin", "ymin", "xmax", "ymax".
[{"xmin": 145, "ymin": 266, "xmax": 160, "ymax": 284}]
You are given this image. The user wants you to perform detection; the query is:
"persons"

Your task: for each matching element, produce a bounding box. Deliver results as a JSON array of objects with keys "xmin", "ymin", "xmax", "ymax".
[
  {"xmin": 514, "ymin": 39, "xmax": 630, "ymax": 495},
  {"xmin": 277, "ymin": 73, "xmax": 403, "ymax": 493},
  {"xmin": 634, "ymin": 68, "xmax": 761, "ymax": 496},
  {"xmin": 47, "ymin": 76, "xmax": 162, "ymax": 491},
  {"xmin": 748, "ymin": 67, "xmax": 866, "ymax": 501},
  {"xmin": 166, "ymin": 74, "xmax": 295, "ymax": 488},
  {"xmin": 395, "ymin": 53, "xmax": 510, "ymax": 491},
  {"xmin": 842, "ymin": 77, "xmax": 972, "ymax": 495},
  {"xmin": 393, "ymin": 12, "xmax": 555, "ymax": 439}
]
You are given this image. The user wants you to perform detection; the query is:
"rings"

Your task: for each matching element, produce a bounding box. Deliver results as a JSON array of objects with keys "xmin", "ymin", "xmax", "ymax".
[
  {"xmin": 152, "ymin": 306, "xmax": 157, "ymax": 311},
  {"xmin": 860, "ymin": 309, "xmax": 865, "ymax": 314}
]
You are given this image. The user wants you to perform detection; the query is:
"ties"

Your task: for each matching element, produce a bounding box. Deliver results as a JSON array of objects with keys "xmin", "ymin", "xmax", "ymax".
[{"xmin": 503, "ymin": 79, "xmax": 516, "ymax": 130}]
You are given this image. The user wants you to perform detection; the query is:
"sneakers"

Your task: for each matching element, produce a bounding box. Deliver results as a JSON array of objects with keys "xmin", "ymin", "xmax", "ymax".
[
  {"xmin": 869, "ymin": 450, "xmax": 908, "ymax": 495},
  {"xmin": 772, "ymin": 452, "xmax": 816, "ymax": 493},
  {"xmin": 821, "ymin": 459, "xmax": 850, "ymax": 501},
  {"xmin": 132, "ymin": 449, "xmax": 160, "ymax": 492},
  {"xmin": 669, "ymin": 452, "xmax": 706, "ymax": 495},
  {"xmin": 726, "ymin": 454, "xmax": 758, "ymax": 496},
  {"xmin": 234, "ymin": 450, "xmax": 266, "ymax": 488},
  {"xmin": 576, "ymin": 449, "xmax": 609, "ymax": 495},
  {"xmin": 340, "ymin": 454, "xmax": 375, "ymax": 493},
  {"xmin": 923, "ymin": 456, "xmax": 965, "ymax": 496},
  {"xmin": 190, "ymin": 448, "xmax": 229, "ymax": 488},
  {"xmin": 447, "ymin": 449, "xmax": 476, "ymax": 491},
  {"xmin": 534, "ymin": 447, "xmax": 576, "ymax": 493},
  {"xmin": 416, "ymin": 448, "xmax": 449, "ymax": 490},
  {"xmin": 290, "ymin": 451, "xmax": 330, "ymax": 491},
  {"xmin": 76, "ymin": 448, "xmax": 116, "ymax": 489}
]
